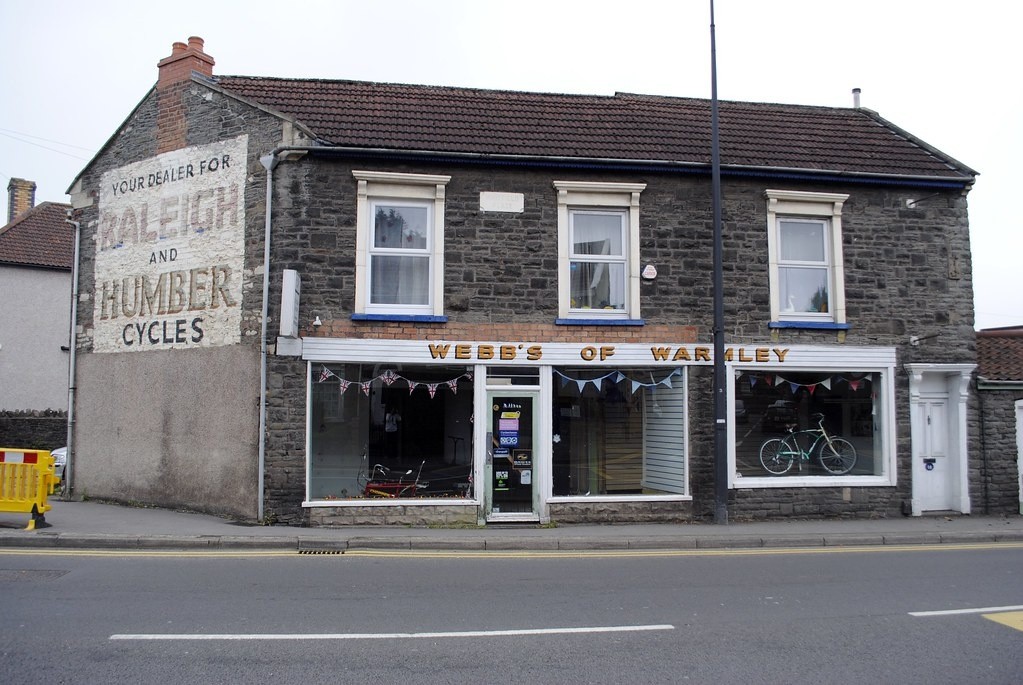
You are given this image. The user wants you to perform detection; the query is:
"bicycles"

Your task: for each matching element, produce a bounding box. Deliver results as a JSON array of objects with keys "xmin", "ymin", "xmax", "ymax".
[{"xmin": 761, "ymin": 412, "xmax": 857, "ymax": 476}]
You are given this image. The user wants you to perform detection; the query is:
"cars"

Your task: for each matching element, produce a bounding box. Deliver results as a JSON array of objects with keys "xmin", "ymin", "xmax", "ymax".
[
  {"xmin": 736, "ymin": 400, "xmax": 747, "ymax": 421},
  {"xmin": 763, "ymin": 399, "xmax": 799, "ymax": 432},
  {"xmin": 49, "ymin": 446, "xmax": 70, "ymax": 478}
]
